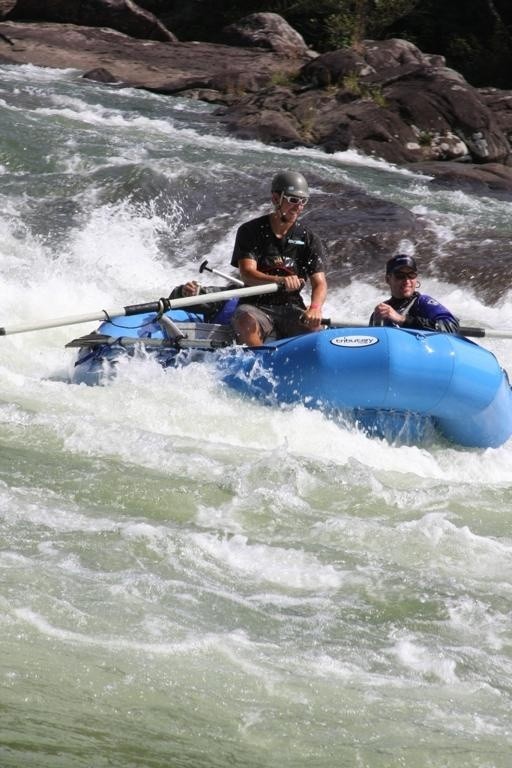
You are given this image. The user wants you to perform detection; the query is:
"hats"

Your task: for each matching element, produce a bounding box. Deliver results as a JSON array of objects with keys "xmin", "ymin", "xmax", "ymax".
[{"xmin": 386, "ymin": 255, "xmax": 417, "ymax": 273}]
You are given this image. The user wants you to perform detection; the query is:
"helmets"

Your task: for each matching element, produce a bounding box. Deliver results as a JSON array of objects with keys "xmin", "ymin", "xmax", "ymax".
[{"xmin": 271, "ymin": 171, "xmax": 310, "ymax": 199}]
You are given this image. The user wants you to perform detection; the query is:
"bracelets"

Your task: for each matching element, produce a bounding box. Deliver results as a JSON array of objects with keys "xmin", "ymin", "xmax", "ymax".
[{"xmin": 309, "ymin": 304, "xmax": 321, "ymax": 310}]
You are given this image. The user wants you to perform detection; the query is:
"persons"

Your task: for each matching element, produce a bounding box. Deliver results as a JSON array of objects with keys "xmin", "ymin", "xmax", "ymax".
[
  {"xmin": 231, "ymin": 171, "xmax": 328, "ymax": 346},
  {"xmin": 369, "ymin": 255, "xmax": 460, "ymax": 334},
  {"xmin": 168, "ymin": 281, "xmax": 242, "ymax": 325}
]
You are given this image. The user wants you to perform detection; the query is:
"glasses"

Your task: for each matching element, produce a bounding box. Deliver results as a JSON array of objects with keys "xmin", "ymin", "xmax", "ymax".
[
  {"xmin": 392, "ymin": 270, "xmax": 418, "ymax": 280},
  {"xmin": 284, "ymin": 195, "xmax": 308, "ymax": 206}
]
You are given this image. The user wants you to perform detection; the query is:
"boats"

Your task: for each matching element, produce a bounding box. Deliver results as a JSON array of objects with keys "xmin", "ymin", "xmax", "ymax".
[{"xmin": 74, "ymin": 297, "xmax": 510, "ymax": 449}]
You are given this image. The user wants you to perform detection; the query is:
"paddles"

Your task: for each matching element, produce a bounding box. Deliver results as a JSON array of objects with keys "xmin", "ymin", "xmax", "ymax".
[{"xmin": 65, "ymin": 334, "xmax": 230, "ymax": 347}]
